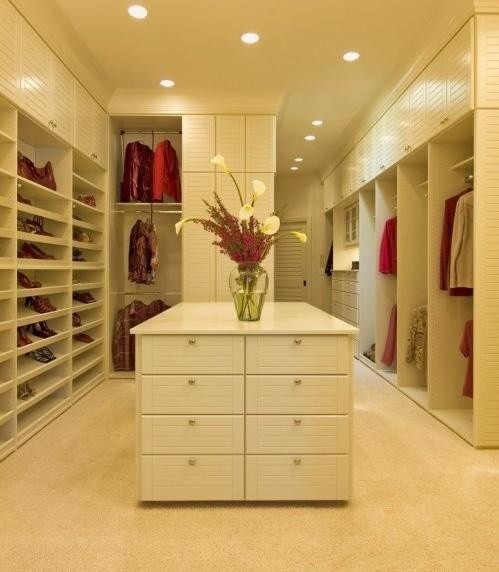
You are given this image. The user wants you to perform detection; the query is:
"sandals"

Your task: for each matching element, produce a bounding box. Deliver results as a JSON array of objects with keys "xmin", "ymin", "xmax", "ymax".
[
  {"xmin": 17, "ymin": 385, "xmax": 31, "ymax": 401},
  {"xmin": 24, "ymin": 296, "xmax": 50, "ymax": 313},
  {"xmin": 36, "ymin": 295, "xmax": 57, "ymax": 311},
  {"xmin": 27, "ymin": 379, "xmax": 37, "ymax": 398}
]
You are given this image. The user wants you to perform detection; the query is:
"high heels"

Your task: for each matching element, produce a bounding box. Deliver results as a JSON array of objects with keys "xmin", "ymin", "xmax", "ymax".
[
  {"xmin": 17, "ymin": 330, "xmax": 26, "ymax": 347},
  {"xmin": 39, "ymin": 321, "xmax": 59, "ymax": 336},
  {"xmin": 19, "ymin": 325, "xmax": 34, "ymax": 344},
  {"xmin": 24, "ymin": 215, "xmax": 54, "ymax": 237},
  {"xmin": 26, "ymin": 324, "xmax": 51, "ymax": 339},
  {"xmin": 23, "ymin": 241, "xmax": 48, "ymax": 260},
  {"xmin": 73, "ymin": 249, "xmax": 86, "ymax": 261},
  {"xmin": 40, "ymin": 346, "xmax": 56, "ymax": 360},
  {"xmin": 73, "ymin": 314, "xmax": 80, "ymax": 326},
  {"xmin": 18, "ymin": 270, "xmax": 42, "ymax": 288},
  {"xmin": 30, "ymin": 242, "xmax": 57, "ymax": 260},
  {"xmin": 25, "ymin": 351, "xmax": 51, "ymax": 364}
]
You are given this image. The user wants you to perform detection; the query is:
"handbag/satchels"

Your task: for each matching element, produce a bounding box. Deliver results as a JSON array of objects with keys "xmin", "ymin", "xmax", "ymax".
[
  {"xmin": 77, "ymin": 194, "xmax": 96, "ymax": 208},
  {"xmin": 17, "ymin": 151, "xmax": 58, "ymax": 192}
]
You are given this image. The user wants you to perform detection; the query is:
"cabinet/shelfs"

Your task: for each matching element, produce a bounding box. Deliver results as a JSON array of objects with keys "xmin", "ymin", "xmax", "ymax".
[
  {"xmin": 0, "ymin": 0, "xmax": 21, "ymax": 103},
  {"xmin": 16, "ymin": 10, "xmax": 74, "ymax": 146},
  {"xmin": 428, "ymin": 110, "xmax": 498, "ymax": 447},
  {"xmin": 323, "ymin": 210, "xmax": 333, "ymax": 312},
  {"xmin": 109, "ymin": 114, "xmax": 182, "ymax": 379},
  {"xmin": 377, "ymin": 104, "xmax": 395, "ymax": 183},
  {"xmin": 427, "ymin": 14, "xmax": 498, "ymax": 140},
  {"xmin": 396, "ymin": 143, "xmax": 428, "ymax": 411},
  {"xmin": 394, "ymin": 65, "xmax": 427, "ymax": 160},
  {"xmin": 182, "ymin": 114, "xmax": 274, "ymax": 301},
  {"xmin": 75, "ymin": 76, "xmax": 108, "ymax": 170},
  {"xmin": 359, "ymin": 176, "xmax": 377, "ymax": 370},
  {"xmin": 377, "ymin": 160, "xmax": 397, "ymax": 383},
  {"xmin": 1, "ymin": 95, "xmax": 110, "ymax": 464},
  {"xmin": 323, "ymin": 150, "xmax": 356, "ymax": 213},
  {"xmin": 357, "ymin": 120, "xmax": 377, "ymax": 189},
  {"xmin": 331, "ymin": 272, "xmax": 359, "ymax": 355},
  {"xmin": 127, "ymin": 302, "xmax": 360, "ymax": 503}
]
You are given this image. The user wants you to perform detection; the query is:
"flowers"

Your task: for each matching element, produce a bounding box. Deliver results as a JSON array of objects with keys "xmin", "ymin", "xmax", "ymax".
[{"xmin": 173, "ymin": 155, "xmax": 307, "ymax": 267}]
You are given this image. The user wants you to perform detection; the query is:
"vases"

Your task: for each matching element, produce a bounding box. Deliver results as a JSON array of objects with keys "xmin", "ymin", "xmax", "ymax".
[{"xmin": 228, "ymin": 264, "xmax": 269, "ymax": 322}]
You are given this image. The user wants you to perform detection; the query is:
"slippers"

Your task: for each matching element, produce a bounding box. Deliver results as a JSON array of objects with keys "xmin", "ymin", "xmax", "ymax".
[
  {"xmin": 75, "ymin": 333, "xmax": 94, "ymax": 342},
  {"xmin": 73, "ymin": 291, "xmax": 98, "ymax": 303},
  {"xmin": 73, "ymin": 228, "xmax": 93, "ymax": 243}
]
